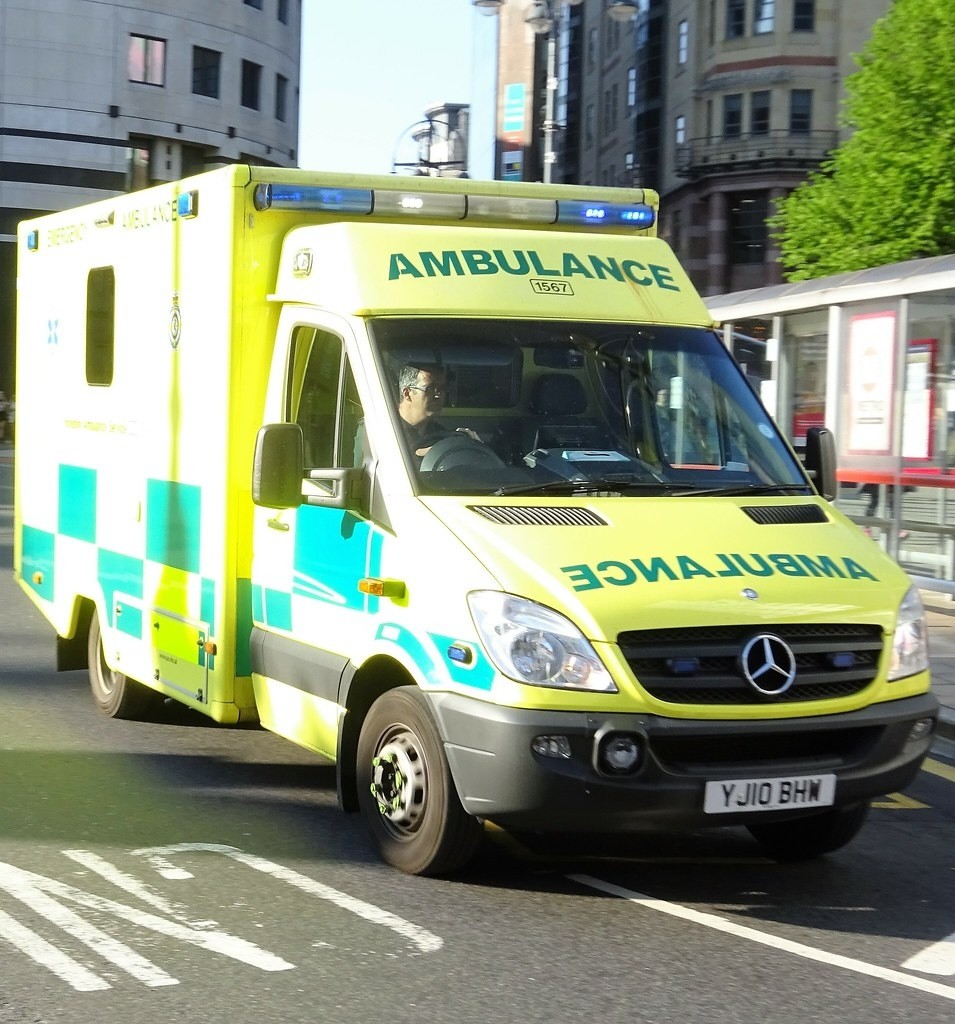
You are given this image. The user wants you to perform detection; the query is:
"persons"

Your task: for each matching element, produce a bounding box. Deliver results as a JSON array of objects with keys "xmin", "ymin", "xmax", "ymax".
[{"xmin": 352, "ymin": 362, "xmax": 484, "ymax": 474}]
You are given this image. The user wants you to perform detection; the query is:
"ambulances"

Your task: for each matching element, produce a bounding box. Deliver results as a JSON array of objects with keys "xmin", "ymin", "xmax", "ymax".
[{"xmin": 9, "ymin": 161, "xmax": 941, "ymax": 879}]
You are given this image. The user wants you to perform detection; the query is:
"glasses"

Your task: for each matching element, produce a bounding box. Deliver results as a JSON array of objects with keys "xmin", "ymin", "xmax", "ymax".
[{"xmin": 407, "ymin": 384, "xmax": 446, "ymax": 395}]
